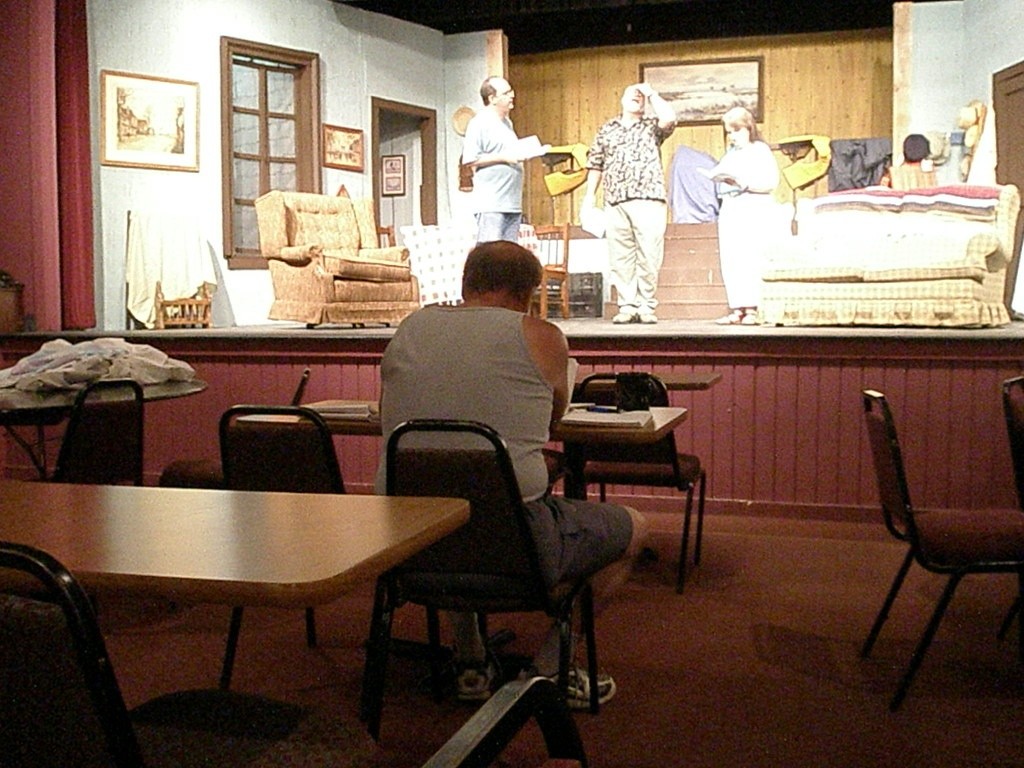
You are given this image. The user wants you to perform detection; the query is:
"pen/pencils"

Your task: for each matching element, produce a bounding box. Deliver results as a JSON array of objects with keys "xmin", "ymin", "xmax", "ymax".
[{"xmin": 586, "ymin": 406, "xmax": 619, "ymax": 413}]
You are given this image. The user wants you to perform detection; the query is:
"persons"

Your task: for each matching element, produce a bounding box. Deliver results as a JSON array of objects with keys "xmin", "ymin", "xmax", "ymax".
[
  {"xmin": 579, "ymin": 82, "xmax": 677, "ymax": 322},
  {"xmin": 461, "ymin": 73, "xmax": 537, "ymax": 247},
  {"xmin": 711, "ymin": 107, "xmax": 781, "ymax": 325},
  {"xmin": 372, "ymin": 240, "xmax": 650, "ymax": 707}
]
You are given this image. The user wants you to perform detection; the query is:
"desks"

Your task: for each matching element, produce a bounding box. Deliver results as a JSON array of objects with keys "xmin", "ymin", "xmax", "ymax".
[
  {"xmin": 299, "ymin": 400, "xmax": 689, "ymax": 500},
  {"xmin": 0, "ymin": 379, "xmax": 208, "ymax": 482},
  {"xmin": 575, "ymin": 372, "xmax": 722, "ymax": 391},
  {"xmin": 537, "ymin": 238, "xmax": 612, "ymax": 319},
  {"xmin": 0, "ymin": 479, "xmax": 472, "ymax": 610}
]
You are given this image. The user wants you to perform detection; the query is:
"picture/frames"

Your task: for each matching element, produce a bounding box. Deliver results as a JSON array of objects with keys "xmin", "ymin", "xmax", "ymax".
[
  {"xmin": 638, "ymin": 56, "xmax": 766, "ymax": 127},
  {"xmin": 321, "ymin": 123, "xmax": 364, "ymax": 173},
  {"xmin": 99, "ymin": 70, "xmax": 200, "ymax": 173},
  {"xmin": 381, "ymin": 155, "xmax": 406, "ymax": 196}
]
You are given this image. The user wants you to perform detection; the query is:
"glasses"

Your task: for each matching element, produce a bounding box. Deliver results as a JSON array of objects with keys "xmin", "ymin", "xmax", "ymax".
[{"xmin": 495, "ymin": 89, "xmax": 512, "ymax": 97}]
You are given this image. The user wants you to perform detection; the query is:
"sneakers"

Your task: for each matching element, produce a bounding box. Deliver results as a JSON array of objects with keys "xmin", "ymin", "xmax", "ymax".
[
  {"xmin": 457, "ymin": 663, "xmax": 502, "ymax": 700},
  {"xmin": 550, "ymin": 665, "xmax": 617, "ymax": 708}
]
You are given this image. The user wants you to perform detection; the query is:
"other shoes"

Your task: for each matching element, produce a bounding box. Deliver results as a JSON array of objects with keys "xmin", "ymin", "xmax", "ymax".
[
  {"xmin": 742, "ymin": 313, "xmax": 764, "ymax": 326},
  {"xmin": 613, "ymin": 314, "xmax": 635, "ymax": 324},
  {"xmin": 717, "ymin": 312, "xmax": 742, "ymax": 325},
  {"xmin": 640, "ymin": 314, "xmax": 659, "ymax": 324}
]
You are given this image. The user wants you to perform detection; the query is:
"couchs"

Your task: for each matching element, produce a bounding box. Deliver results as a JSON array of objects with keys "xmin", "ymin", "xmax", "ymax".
[
  {"xmin": 254, "ymin": 190, "xmax": 421, "ymax": 328},
  {"xmin": 755, "ymin": 183, "xmax": 1021, "ymax": 328}
]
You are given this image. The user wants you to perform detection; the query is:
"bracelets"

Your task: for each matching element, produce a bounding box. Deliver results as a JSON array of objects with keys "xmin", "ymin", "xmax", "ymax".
[{"xmin": 648, "ymin": 90, "xmax": 658, "ymax": 102}]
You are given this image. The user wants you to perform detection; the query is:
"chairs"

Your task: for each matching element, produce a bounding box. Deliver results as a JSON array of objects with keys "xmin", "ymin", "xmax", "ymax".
[
  {"xmin": 996, "ymin": 377, "xmax": 1023, "ymax": 639},
  {"xmin": 0, "ymin": 538, "xmax": 151, "ymax": 768},
  {"xmin": 860, "ymin": 389, "xmax": 1024, "ymax": 712},
  {"xmin": 51, "ymin": 378, "xmax": 145, "ymax": 486},
  {"xmin": 160, "ymin": 367, "xmax": 310, "ymax": 491},
  {"xmin": 564, "ymin": 371, "xmax": 707, "ymax": 595},
  {"xmin": 219, "ymin": 404, "xmax": 441, "ymax": 696},
  {"xmin": 532, "ymin": 222, "xmax": 571, "ymax": 319},
  {"xmin": 123, "ymin": 210, "xmax": 218, "ymax": 330},
  {"xmin": 368, "ymin": 419, "xmax": 598, "ymax": 741}
]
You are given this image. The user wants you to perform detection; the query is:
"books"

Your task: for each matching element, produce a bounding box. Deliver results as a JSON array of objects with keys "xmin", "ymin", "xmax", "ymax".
[{"xmin": 561, "ymin": 410, "xmax": 652, "ymax": 428}]
